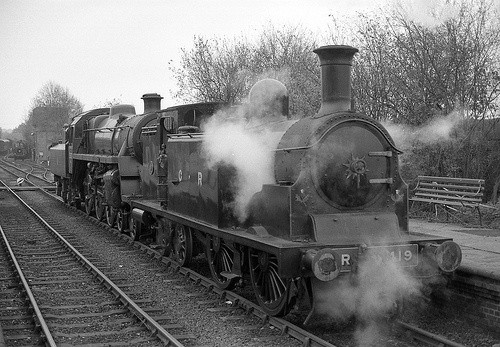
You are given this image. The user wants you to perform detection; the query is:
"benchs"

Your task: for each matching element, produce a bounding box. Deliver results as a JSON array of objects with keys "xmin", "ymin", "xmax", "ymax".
[{"xmin": 406, "ymin": 175, "xmax": 485, "ymax": 228}]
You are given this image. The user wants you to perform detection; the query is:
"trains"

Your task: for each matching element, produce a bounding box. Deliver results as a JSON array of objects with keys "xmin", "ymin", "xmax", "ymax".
[
  {"xmin": 14, "ymin": 140, "xmax": 27, "ymax": 161},
  {"xmin": 49, "ymin": 46, "xmax": 462, "ymax": 330}
]
